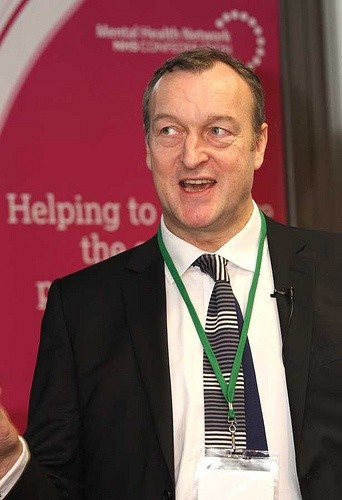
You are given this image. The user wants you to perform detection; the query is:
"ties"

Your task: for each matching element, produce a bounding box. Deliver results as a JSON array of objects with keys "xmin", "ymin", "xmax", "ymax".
[{"xmin": 189, "ymin": 253, "xmax": 269, "ymax": 458}]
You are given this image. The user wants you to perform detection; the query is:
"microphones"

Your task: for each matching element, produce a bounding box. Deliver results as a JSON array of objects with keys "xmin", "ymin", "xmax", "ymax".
[{"xmin": 275, "ymin": 287, "xmax": 294, "ymax": 299}]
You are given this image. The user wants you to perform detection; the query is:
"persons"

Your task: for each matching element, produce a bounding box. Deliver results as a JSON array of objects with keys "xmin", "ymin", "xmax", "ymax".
[{"xmin": 0, "ymin": 47, "xmax": 340, "ymax": 500}]
